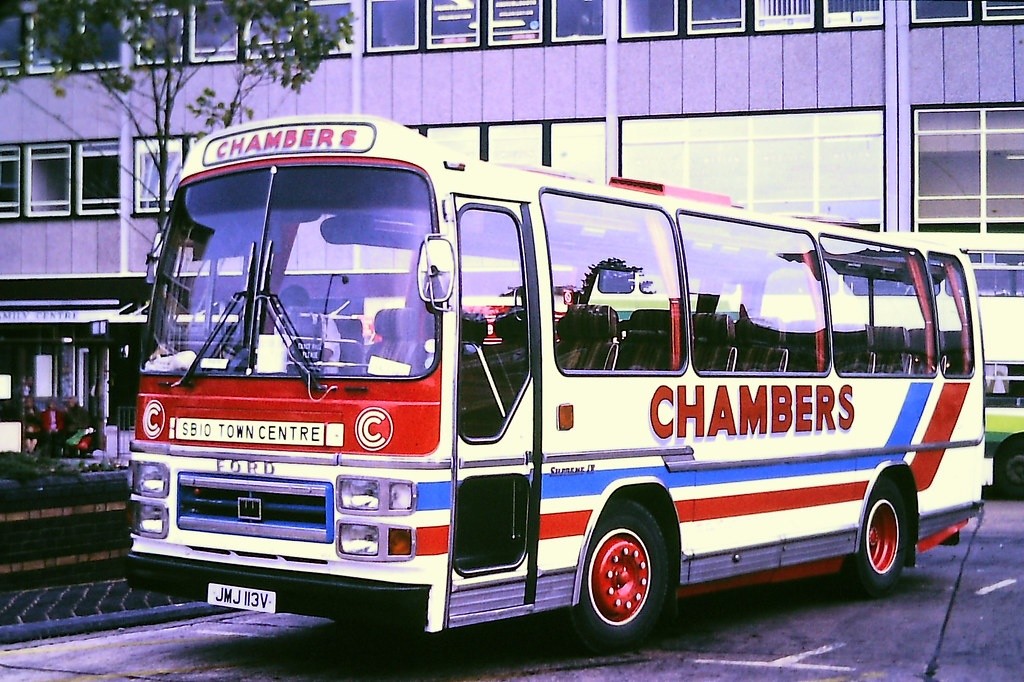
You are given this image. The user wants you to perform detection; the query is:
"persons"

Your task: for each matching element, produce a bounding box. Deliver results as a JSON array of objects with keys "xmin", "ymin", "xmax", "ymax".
[
  {"xmin": 41, "ymin": 397, "xmax": 65, "ymax": 457},
  {"xmin": 24, "ymin": 397, "xmax": 41, "ymax": 453},
  {"xmin": 61, "ymin": 396, "xmax": 92, "ymax": 457},
  {"xmin": 277, "ymin": 285, "xmax": 342, "ymax": 376}
]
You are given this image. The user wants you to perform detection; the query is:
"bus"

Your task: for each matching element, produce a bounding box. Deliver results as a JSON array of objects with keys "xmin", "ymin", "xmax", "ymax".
[
  {"xmin": 526, "ymin": 198, "xmax": 1024, "ymax": 513},
  {"xmin": 126, "ymin": 116, "xmax": 986, "ymax": 656}
]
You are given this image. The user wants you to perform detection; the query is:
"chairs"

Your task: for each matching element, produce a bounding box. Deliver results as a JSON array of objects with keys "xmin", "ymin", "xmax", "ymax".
[{"xmin": 242, "ymin": 289, "xmax": 971, "ymax": 438}]
